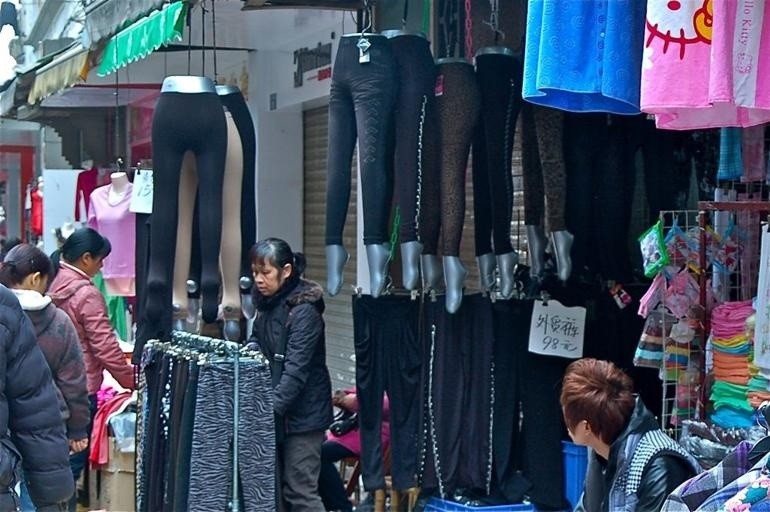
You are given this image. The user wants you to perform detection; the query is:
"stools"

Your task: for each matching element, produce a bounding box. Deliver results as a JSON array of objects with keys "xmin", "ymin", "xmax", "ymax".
[{"xmin": 339, "ymin": 459, "xmax": 360, "ymax": 495}]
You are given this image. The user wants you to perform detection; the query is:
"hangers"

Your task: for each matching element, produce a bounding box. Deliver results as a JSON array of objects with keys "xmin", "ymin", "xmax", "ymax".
[
  {"xmin": 351, "ymin": 270, "xmax": 529, "ymax": 301},
  {"xmin": 143, "ymin": 330, "xmax": 273, "ymax": 368}
]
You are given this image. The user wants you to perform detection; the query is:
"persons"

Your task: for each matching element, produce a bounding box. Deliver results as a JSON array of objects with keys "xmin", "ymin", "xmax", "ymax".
[
  {"xmin": 326, "ymin": 28, "xmax": 575, "ymax": 315},
  {"xmin": 43, "ymin": 227, "xmax": 136, "ymax": 512},
  {"xmin": 317, "ymin": 385, "xmax": 393, "ymax": 511},
  {"xmin": 145, "ymin": 75, "xmax": 255, "ymax": 339},
  {"xmin": 238, "ymin": 238, "xmax": 336, "ymax": 510},
  {"xmin": 51, "ymin": 223, "xmax": 78, "ymax": 247},
  {"xmin": 1, "ymin": 243, "xmax": 90, "ymax": 511},
  {"xmin": 0, "ymin": 283, "xmax": 78, "ymax": 512},
  {"xmin": 559, "ymin": 355, "xmax": 704, "ymax": 510},
  {"xmin": 86, "ymin": 173, "xmax": 137, "ymax": 298},
  {"xmin": 32, "ymin": 183, "xmax": 43, "ymax": 235}
]
